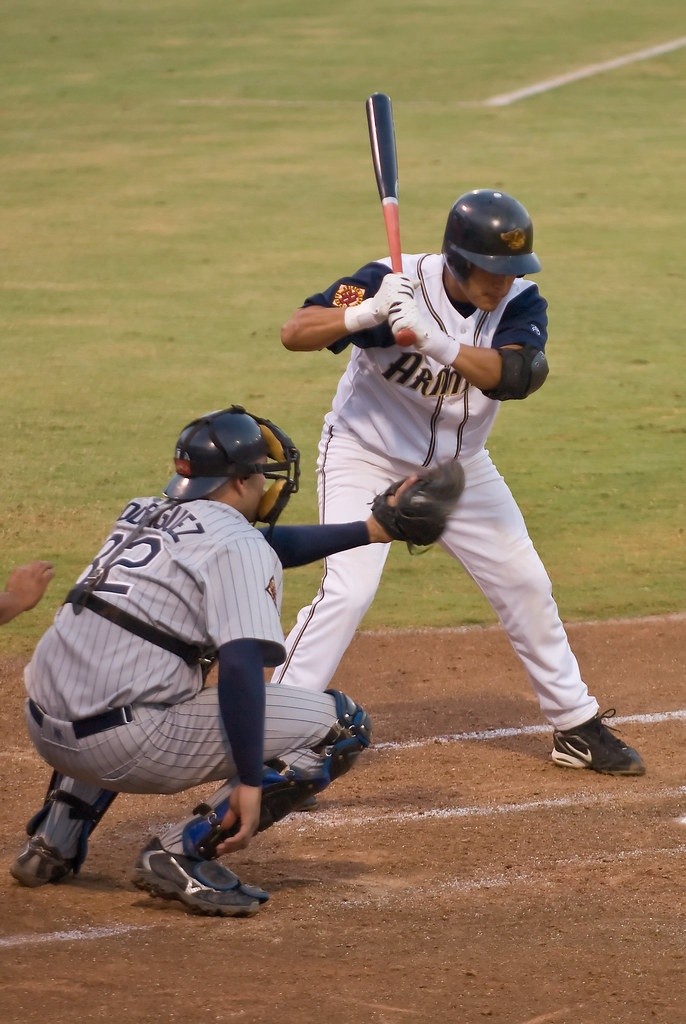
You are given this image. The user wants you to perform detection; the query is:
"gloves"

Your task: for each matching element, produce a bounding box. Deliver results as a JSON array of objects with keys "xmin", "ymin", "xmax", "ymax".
[
  {"xmin": 344, "ymin": 272, "xmax": 424, "ymax": 332},
  {"xmin": 388, "ymin": 299, "xmax": 460, "ymax": 368}
]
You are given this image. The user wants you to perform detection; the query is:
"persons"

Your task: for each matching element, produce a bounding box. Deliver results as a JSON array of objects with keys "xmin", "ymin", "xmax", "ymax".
[
  {"xmin": 0, "ymin": 561, "xmax": 57, "ymax": 626},
  {"xmin": 269, "ymin": 188, "xmax": 645, "ymax": 811},
  {"xmin": 8, "ymin": 407, "xmax": 464, "ymax": 918}
]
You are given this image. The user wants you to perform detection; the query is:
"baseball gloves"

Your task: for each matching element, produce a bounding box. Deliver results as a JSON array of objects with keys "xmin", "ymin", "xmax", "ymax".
[{"xmin": 369, "ymin": 460, "xmax": 464, "ymax": 550}]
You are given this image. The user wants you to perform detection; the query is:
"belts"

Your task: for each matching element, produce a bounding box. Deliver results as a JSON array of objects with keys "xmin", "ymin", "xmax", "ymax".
[{"xmin": 28, "ymin": 697, "xmax": 133, "ymax": 740}]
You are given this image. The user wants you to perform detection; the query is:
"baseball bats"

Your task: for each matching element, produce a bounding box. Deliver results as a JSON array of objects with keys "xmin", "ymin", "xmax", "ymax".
[{"xmin": 365, "ymin": 91, "xmax": 418, "ymax": 348}]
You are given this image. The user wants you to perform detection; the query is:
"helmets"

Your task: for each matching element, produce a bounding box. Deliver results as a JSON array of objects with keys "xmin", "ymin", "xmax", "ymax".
[
  {"xmin": 166, "ymin": 406, "xmax": 266, "ymax": 502},
  {"xmin": 440, "ymin": 190, "xmax": 541, "ymax": 283}
]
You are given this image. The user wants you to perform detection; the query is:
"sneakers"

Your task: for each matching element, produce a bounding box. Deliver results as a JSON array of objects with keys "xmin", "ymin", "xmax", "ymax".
[
  {"xmin": 132, "ymin": 833, "xmax": 270, "ymax": 917},
  {"xmin": 550, "ymin": 707, "xmax": 645, "ymax": 775},
  {"xmin": 9, "ymin": 836, "xmax": 73, "ymax": 889}
]
